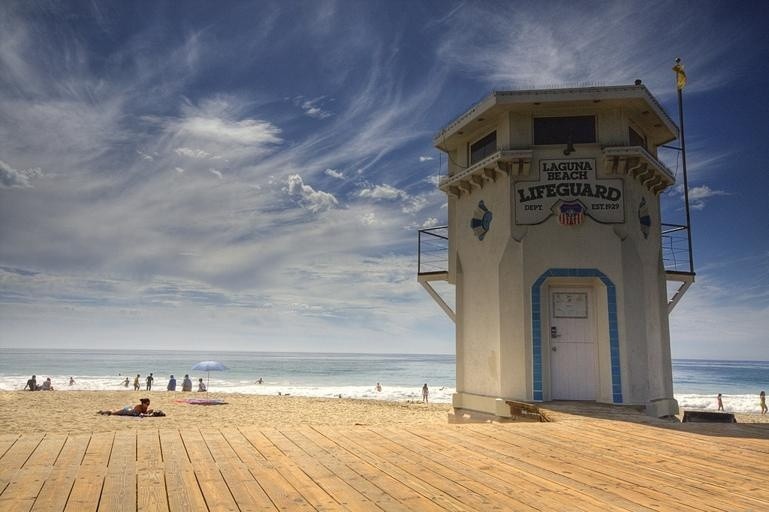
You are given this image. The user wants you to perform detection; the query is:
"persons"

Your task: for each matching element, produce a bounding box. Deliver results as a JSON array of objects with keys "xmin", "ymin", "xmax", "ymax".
[
  {"xmin": 375, "ymin": 382, "xmax": 381, "ymax": 392},
  {"xmin": 717, "ymin": 393, "xmax": 725, "ymax": 411},
  {"xmin": 254, "ymin": 377, "xmax": 264, "ymax": 384},
  {"xmin": 95, "ymin": 398, "xmax": 155, "ymax": 417},
  {"xmin": 120, "ymin": 373, "xmax": 206, "ymax": 393},
  {"xmin": 23, "ymin": 375, "xmax": 43, "ymax": 391},
  {"xmin": 42, "ymin": 378, "xmax": 54, "ymax": 391},
  {"xmin": 69, "ymin": 376, "xmax": 75, "ymax": 386},
  {"xmin": 422, "ymin": 384, "xmax": 429, "ymax": 404},
  {"xmin": 760, "ymin": 391, "xmax": 768, "ymax": 416}
]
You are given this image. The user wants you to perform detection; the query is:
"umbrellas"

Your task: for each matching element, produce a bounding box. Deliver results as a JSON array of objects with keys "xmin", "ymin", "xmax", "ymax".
[{"xmin": 191, "ymin": 360, "xmax": 230, "ymax": 403}]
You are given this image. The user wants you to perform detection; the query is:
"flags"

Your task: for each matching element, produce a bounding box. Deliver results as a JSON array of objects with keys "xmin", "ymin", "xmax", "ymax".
[{"xmin": 672, "ymin": 63, "xmax": 687, "ymax": 91}]
